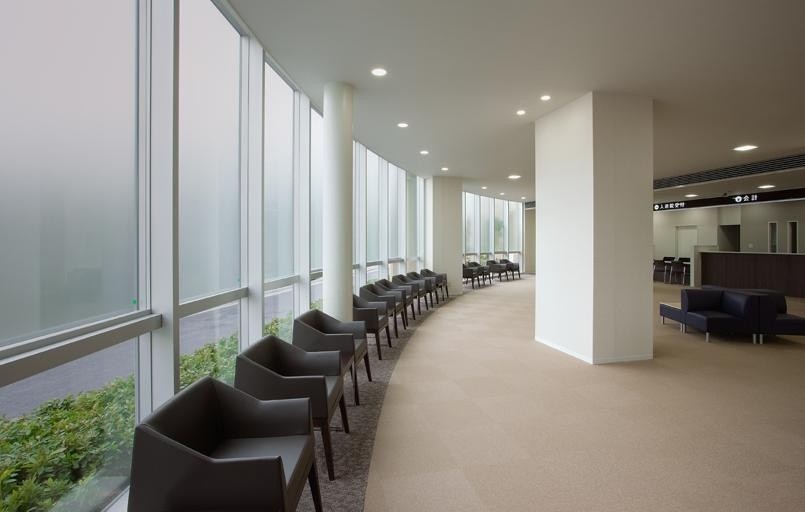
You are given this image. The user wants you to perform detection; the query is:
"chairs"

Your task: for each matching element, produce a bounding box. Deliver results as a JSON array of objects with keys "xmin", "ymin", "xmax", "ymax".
[{"xmin": 652, "ymin": 256, "xmax": 805, "ymax": 345}]
